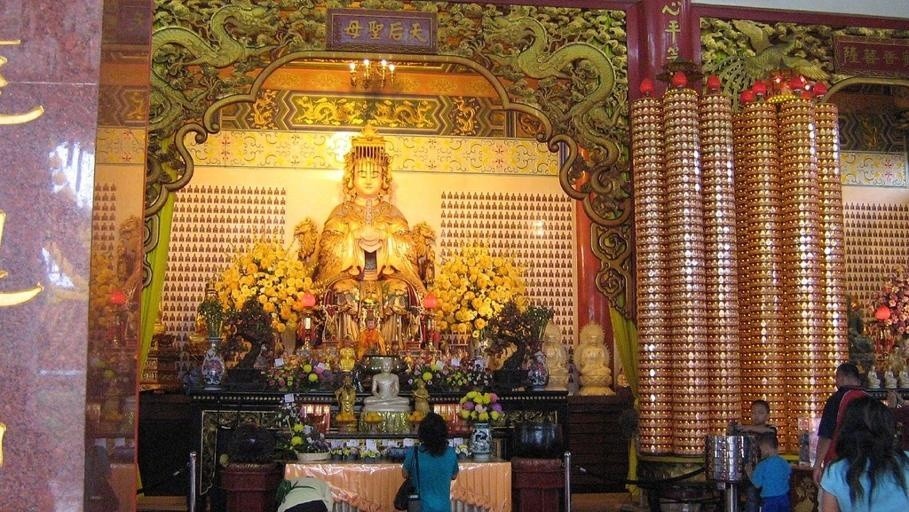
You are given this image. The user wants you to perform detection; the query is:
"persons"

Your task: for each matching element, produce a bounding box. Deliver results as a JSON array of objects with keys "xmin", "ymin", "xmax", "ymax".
[
  {"xmin": 542, "ymin": 321, "xmax": 568, "ymax": 376},
  {"xmin": 580, "ymin": 325, "xmax": 612, "ymax": 376},
  {"xmin": 728, "ymin": 399, "xmax": 791, "ymax": 512},
  {"xmin": 402, "ymin": 414, "xmax": 458, "ymax": 512},
  {"xmin": 335, "ymin": 376, "xmax": 356, "ymax": 417},
  {"xmin": 318, "ymin": 145, "xmax": 428, "ymax": 371},
  {"xmin": 414, "ymin": 380, "xmax": 430, "ymax": 412},
  {"xmin": 278, "ymin": 478, "xmax": 334, "ymax": 512},
  {"xmin": 364, "ymin": 360, "xmax": 409, "ymax": 406},
  {"xmin": 812, "ymin": 363, "xmax": 908, "ymax": 512}
]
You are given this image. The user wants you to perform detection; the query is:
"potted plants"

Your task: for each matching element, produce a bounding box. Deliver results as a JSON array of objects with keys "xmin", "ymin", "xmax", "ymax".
[
  {"xmin": 524, "ymin": 306, "xmax": 549, "ymax": 388},
  {"xmin": 484, "ymin": 302, "xmax": 528, "ymax": 392},
  {"xmin": 220, "ymin": 295, "xmax": 273, "ymax": 392},
  {"xmin": 197, "ymin": 296, "xmax": 225, "ymax": 392}
]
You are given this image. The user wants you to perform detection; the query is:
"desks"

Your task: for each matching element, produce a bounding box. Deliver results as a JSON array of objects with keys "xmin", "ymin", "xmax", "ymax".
[{"xmin": 138, "ymin": 385, "xmax": 625, "ymax": 512}]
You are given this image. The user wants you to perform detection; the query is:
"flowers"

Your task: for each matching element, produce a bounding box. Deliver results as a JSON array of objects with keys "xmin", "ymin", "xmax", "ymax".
[
  {"xmin": 870, "ymin": 266, "xmax": 909, "ymax": 337},
  {"xmin": 214, "ymin": 229, "xmax": 317, "ymax": 333},
  {"xmin": 429, "ymin": 245, "xmax": 529, "ymax": 339}
]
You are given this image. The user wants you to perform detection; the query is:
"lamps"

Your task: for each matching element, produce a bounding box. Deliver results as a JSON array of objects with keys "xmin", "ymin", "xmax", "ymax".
[{"xmin": 350, "ymin": 59, "xmax": 394, "ymax": 89}]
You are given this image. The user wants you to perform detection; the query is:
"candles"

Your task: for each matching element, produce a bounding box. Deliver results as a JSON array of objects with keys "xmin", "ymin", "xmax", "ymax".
[{"xmin": 305, "ymin": 314, "xmax": 311, "ymax": 330}]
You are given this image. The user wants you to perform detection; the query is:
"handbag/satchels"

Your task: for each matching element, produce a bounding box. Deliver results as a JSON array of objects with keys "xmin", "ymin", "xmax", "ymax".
[
  {"xmin": 394, "ymin": 446, "xmax": 421, "ymax": 512},
  {"xmin": 274, "ymin": 479, "xmax": 314, "ymax": 505}
]
